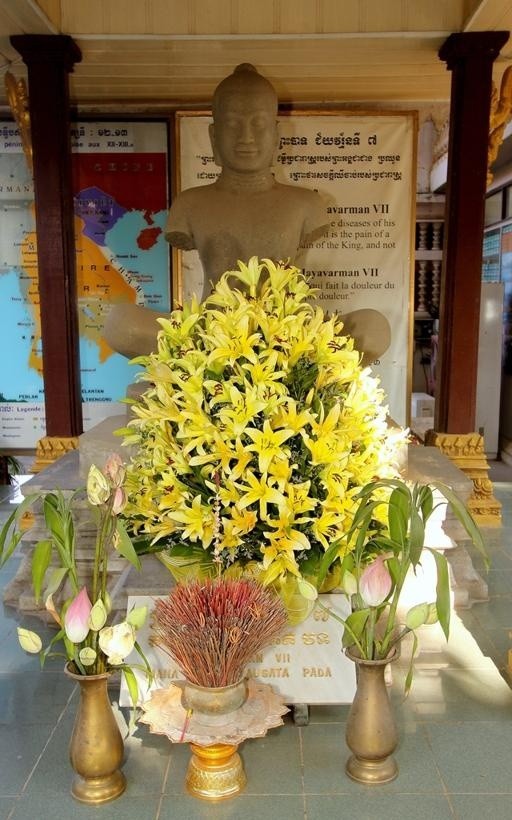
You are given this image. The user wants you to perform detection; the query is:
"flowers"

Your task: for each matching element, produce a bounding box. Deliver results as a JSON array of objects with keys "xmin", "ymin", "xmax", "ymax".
[
  {"xmin": 1, "ymin": 453, "xmax": 164, "ymax": 714},
  {"xmin": 103, "ymin": 252, "xmax": 417, "ymax": 580},
  {"xmin": 296, "ymin": 472, "xmax": 493, "ymax": 705}
]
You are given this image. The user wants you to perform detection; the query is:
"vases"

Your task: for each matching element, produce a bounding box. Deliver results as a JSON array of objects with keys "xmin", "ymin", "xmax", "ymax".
[
  {"xmin": 63, "ymin": 658, "xmax": 129, "ymax": 806},
  {"xmin": 181, "ymin": 668, "xmax": 249, "ymax": 719},
  {"xmin": 345, "ymin": 642, "xmax": 401, "ymax": 786},
  {"xmin": 152, "ymin": 537, "xmax": 344, "ymax": 594}
]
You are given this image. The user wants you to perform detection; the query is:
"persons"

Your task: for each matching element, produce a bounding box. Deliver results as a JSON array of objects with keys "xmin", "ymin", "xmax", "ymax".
[{"xmin": 103, "ymin": 61, "xmax": 392, "ymax": 370}]
